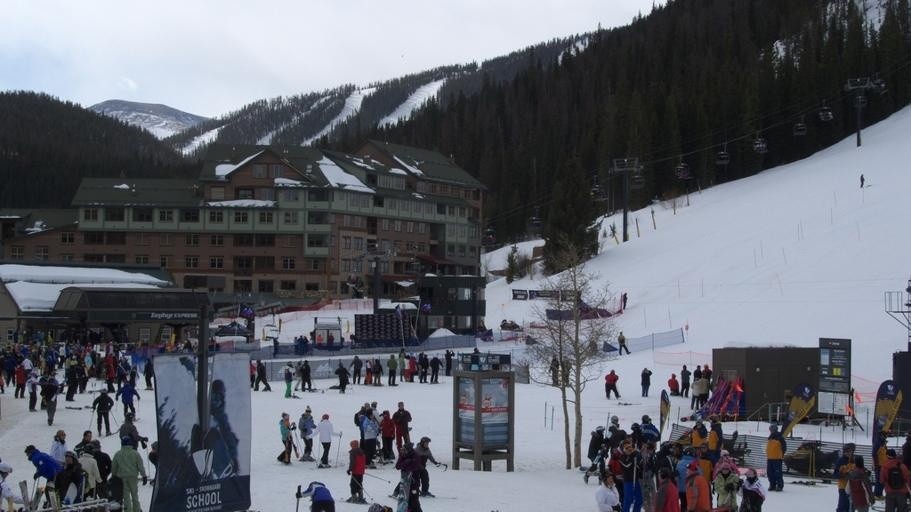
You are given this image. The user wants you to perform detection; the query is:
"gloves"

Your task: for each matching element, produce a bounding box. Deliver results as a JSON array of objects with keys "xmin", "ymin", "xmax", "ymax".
[
  {"xmin": 141, "ymin": 437, "xmax": 148, "ymax": 449},
  {"xmin": 296, "ymin": 493, "xmax": 301, "ymax": 498},
  {"xmin": 347, "ymin": 469, "xmax": 351, "ymax": 475},
  {"xmin": 143, "ymin": 477, "xmax": 147, "ymax": 485},
  {"xmin": 292, "ymin": 426, "xmax": 296, "ymax": 430},
  {"xmin": 34, "ymin": 474, "xmax": 38, "ymax": 480}
]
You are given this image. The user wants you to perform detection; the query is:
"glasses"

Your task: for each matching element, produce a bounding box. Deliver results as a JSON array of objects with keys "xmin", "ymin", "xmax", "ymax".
[
  {"xmin": 212, "ymin": 393, "xmax": 222, "ymax": 399},
  {"xmin": 687, "ymin": 463, "xmax": 692, "ymax": 468}
]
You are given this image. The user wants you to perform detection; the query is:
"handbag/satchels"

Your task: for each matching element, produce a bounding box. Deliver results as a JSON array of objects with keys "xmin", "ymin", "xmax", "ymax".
[{"xmin": 888, "ymin": 467, "xmax": 906, "ymax": 489}]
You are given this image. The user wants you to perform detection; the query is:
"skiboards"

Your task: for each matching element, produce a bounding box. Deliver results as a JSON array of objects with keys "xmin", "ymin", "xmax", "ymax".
[
  {"xmin": 295, "ymin": 387, "xmax": 320, "ymax": 391},
  {"xmin": 388, "ymin": 494, "xmax": 458, "ymax": 500},
  {"xmin": 19, "ymin": 480, "xmax": 62, "ymax": 512},
  {"xmin": 584, "ymin": 427, "xmax": 613, "ymax": 486},
  {"xmin": 339, "ymin": 497, "xmax": 373, "ymax": 505},
  {"xmin": 92, "ymin": 430, "xmax": 120, "ymax": 439}
]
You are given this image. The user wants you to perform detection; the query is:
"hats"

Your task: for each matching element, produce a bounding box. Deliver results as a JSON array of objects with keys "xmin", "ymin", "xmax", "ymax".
[
  {"xmin": 84, "ymin": 439, "xmax": 101, "ymax": 454},
  {"xmin": 64, "ymin": 450, "xmax": 77, "ymax": 457},
  {"xmin": 306, "ymin": 406, "xmax": 311, "ymax": 413},
  {"xmin": 0, "ymin": 463, "xmax": 12, "ymax": 472},
  {"xmin": 721, "ymin": 449, "xmax": 731, "ymax": 469},
  {"xmin": 351, "ymin": 441, "xmax": 358, "ymax": 448},
  {"xmin": 323, "ymin": 415, "xmax": 329, "ymax": 419},
  {"xmin": 25, "ymin": 445, "xmax": 35, "ymax": 453},
  {"xmin": 686, "ymin": 460, "xmax": 700, "ymax": 472},
  {"xmin": 122, "ymin": 436, "xmax": 132, "ymax": 445}
]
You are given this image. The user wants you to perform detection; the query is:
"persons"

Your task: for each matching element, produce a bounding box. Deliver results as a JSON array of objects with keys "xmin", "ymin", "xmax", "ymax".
[
  {"xmin": 249, "ymin": 332, "xmax": 456, "ymax": 399},
  {"xmin": 668, "ymin": 365, "xmax": 691, "ymax": 397},
  {"xmin": 781, "ymin": 389, "xmax": 793, "ymax": 437},
  {"xmin": 585, "ymin": 415, "xmax": 765, "ymax": 512},
  {"xmin": 834, "ymin": 444, "xmax": 875, "ymax": 511},
  {"xmin": 623, "ymin": 292, "xmax": 629, "ymax": 310},
  {"xmin": 619, "ymin": 331, "xmax": 633, "ymax": 356},
  {"xmin": 562, "ymin": 356, "xmax": 574, "ymax": 388},
  {"xmin": 605, "ymin": 370, "xmax": 621, "ymax": 399},
  {"xmin": 767, "ymin": 425, "xmax": 787, "ymax": 492},
  {"xmin": 641, "ymin": 368, "xmax": 652, "ymax": 397},
  {"xmin": 273, "ymin": 400, "xmax": 443, "ymax": 512},
  {"xmin": 691, "ymin": 365, "xmax": 712, "ymax": 411},
  {"xmin": 1, "ymin": 325, "xmax": 195, "ymax": 511},
  {"xmin": 208, "ymin": 379, "xmax": 241, "ymax": 474},
  {"xmin": 872, "ymin": 431, "xmax": 910, "ymax": 512},
  {"xmin": 549, "ymin": 355, "xmax": 561, "ymax": 387}
]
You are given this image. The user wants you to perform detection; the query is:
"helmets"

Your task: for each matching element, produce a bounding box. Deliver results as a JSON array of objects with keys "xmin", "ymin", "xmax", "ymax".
[
  {"xmin": 212, "ymin": 380, "xmax": 225, "ymax": 398},
  {"xmin": 631, "ymin": 415, "xmax": 650, "ymax": 432},
  {"xmin": 126, "ymin": 412, "xmax": 135, "ymax": 419},
  {"xmin": 683, "ymin": 447, "xmax": 697, "ymax": 457},
  {"xmin": 420, "ymin": 437, "xmax": 431, "ymax": 442},
  {"xmin": 596, "ymin": 416, "xmax": 619, "ymax": 431}
]
